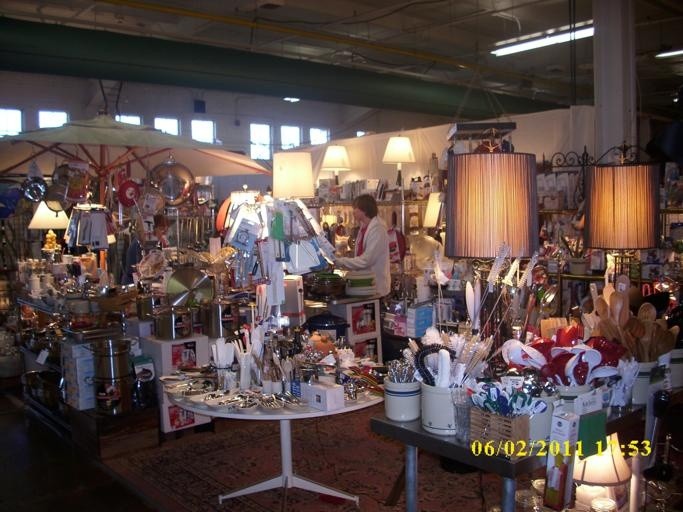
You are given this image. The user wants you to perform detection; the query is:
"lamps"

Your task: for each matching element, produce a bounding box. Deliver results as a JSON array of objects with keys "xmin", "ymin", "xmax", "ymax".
[
  {"xmin": 488, "ymin": 0, "xmax": 593, "ymax": 57},
  {"xmin": 582, "ymin": 163, "xmax": 664, "ymax": 298},
  {"xmin": 573, "ymin": 432, "xmax": 631, "ymax": 485},
  {"xmin": 381, "ymin": 134, "xmax": 414, "ymax": 186},
  {"xmin": 26, "ymin": 201, "xmax": 70, "ymax": 257},
  {"xmin": 271, "ymin": 152, "xmax": 314, "ymax": 200},
  {"xmin": 443, "ymin": 152, "xmax": 540, "ymax": 334}
]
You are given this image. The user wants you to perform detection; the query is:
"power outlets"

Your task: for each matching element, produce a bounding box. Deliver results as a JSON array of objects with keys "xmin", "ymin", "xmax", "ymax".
[{"xmin": 320, "ymin": 145, "xmax": 351, "ymax": 184}]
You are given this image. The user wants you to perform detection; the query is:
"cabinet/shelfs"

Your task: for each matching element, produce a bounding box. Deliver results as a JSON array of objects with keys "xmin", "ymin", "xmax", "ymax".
[
  {"xmin": 473, "ymin": 160, "xmax": 683, "ymax": 315},
  {"xmin": 329, "ymin": 300, "xmax": 385, "ymax": 369},
  {"xmin": 142, "ymin": 334, "xmax": 211, "ymax": 433},
  {"xmin": 14, "ymin": 295, "xmax": 159, "ymax": 459},
  {"xmin": 322, "ymin": 198, "xmax": 433, "ymax": 335}
]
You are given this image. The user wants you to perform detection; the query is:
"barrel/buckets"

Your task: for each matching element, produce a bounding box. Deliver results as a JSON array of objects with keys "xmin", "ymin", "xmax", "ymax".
[
  {"xmin": 418, "ymin": 381, "xmax": 465, "ymax": 438},
  {"xmin": 530, "ymin": 349, "xmax": 683, "ymax": 450}
]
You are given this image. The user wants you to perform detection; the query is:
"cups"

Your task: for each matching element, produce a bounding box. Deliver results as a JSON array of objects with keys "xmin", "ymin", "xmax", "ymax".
[
  {"xmin": 60, "ymin": 292, "xmax": 90, "ymax": 315},
  {"xmin": 451, "ymin": 390, "xmax": 471, "ymax": 442},
  {"xmin": 236, "ymin": 365, "xmax": 252, "ymax": 390},
  {"xmin": 135, "ymin": 297, "xmax": 153, "ymax": 319},
  {"xmin": 522, "ymin": 360, "xmax": 658, "ymax": 449},
  {"xmin": 211, "ymin": 367, "xmax": 232, "ymax": 390},
  {"xmin": 571, "ymin": 257, "xmax": 587, "ymax": 275},
  {"xmin": 419, "ymin": 381, "xmax": 456, "ymax": 436}
]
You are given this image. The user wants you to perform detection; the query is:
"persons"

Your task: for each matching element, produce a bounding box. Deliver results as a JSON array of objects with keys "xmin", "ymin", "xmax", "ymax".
[
  {"xmin": 332, "ymin": 194, "xmax": 392, "ymax": 353},
  {"xmin": 120, "ymin": 214, "xmax": 170, "ymax": 286}
]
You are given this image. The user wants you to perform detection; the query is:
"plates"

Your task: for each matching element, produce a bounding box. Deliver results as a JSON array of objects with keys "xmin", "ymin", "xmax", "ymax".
[{"xmin": 158, "ymin": 375, "xmax": 309, "ymax": 412}]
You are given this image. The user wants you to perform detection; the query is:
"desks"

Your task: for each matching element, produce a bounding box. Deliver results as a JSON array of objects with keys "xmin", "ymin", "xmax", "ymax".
[
  {"xmin": 369, "ymin": 386, "xmax": 647, "ymax": 511},
  {"xmin": 167, "ymin": 365, "xmax": 387, "ymax": 511}
]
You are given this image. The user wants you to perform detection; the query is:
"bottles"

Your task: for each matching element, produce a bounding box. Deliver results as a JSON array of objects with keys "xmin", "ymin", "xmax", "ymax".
[
  {"xmin": 260, "ymin": 325, "xmax": 302, "ymax": 373},
  {"xmin": 24, "ymin": 257, "xmax": 49, "ymax": 293},
  {"xmin": 344, "ymin": 382, "xmax": 356, "ymax": 401},
  {"xmin": 310, "ymin": 331, "xmax": 350, "ymax": 384}
]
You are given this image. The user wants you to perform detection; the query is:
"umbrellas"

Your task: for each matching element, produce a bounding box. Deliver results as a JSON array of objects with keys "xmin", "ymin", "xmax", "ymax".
[{"xmin": 0, "ymin": 114, "xmax": 270, "ymax": 271}]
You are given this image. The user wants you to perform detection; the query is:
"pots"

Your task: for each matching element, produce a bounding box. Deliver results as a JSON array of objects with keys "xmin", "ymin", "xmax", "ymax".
[
  {"xmin": 197, "ymin": 301, "xmax": 241, "ymax": 338},
  {"xmin": 153, "ymin": 306, "xmax": 193, "ymax": 340},
  {"xmin": 19, "ymin": 149, "xmax": 198, "ymax": 213},
  {"xmin": 303, "ymin": 309, "xmax": 350, "ymax": 337},
  {"xmin": 86, "ymin": 337, "xmax": 139, "ymax": 415}
]
